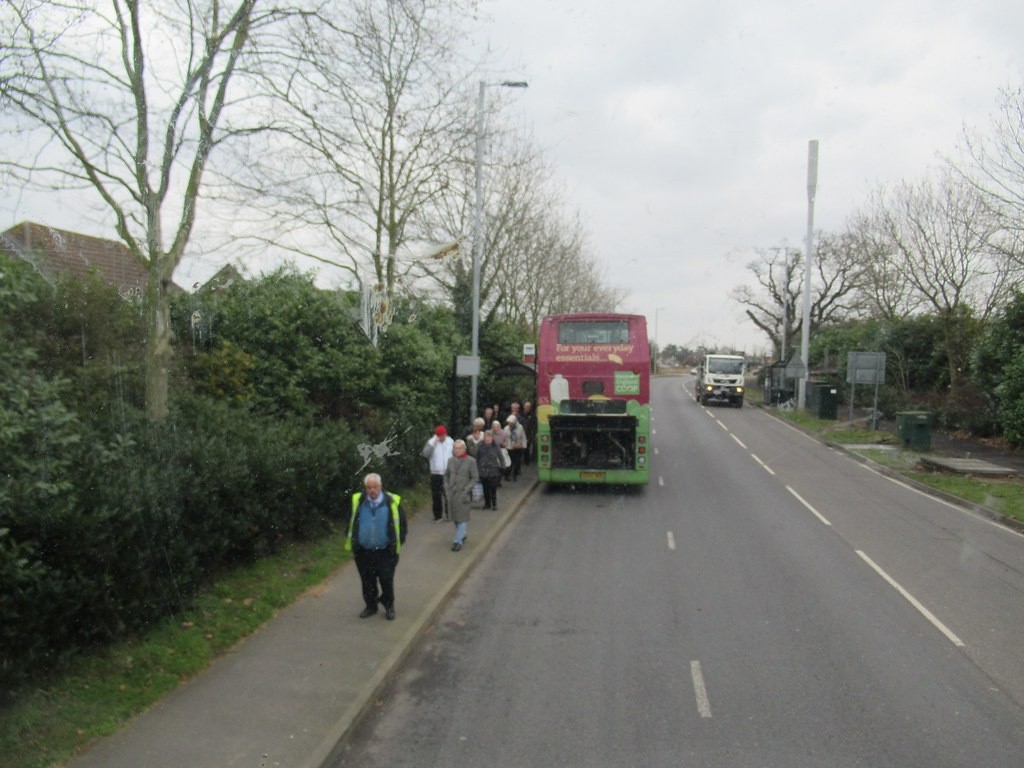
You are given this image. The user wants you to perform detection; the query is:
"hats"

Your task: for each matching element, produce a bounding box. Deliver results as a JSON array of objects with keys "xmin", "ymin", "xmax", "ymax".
[{"xmin": 435, "ymin": 425, "xmax": 447, "ymax": 435}]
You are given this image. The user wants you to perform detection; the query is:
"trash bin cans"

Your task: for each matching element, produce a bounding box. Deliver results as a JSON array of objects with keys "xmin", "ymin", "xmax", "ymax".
[
  {"xmin": 894, "ymin": 410, "xmax": 936, "ymax": 450},
  {"xmin": 813, "ymin": 385, "xmax": 841, "ymax": 419},
  {"xmin": 805, "ymin": 381, "xmax": 828, "ymax": 415}
]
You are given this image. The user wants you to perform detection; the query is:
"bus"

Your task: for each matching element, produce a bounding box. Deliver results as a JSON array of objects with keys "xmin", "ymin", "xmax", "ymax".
[{"xmin": 537, "ymin": 311, "xmax": 651, "ymax": 486}]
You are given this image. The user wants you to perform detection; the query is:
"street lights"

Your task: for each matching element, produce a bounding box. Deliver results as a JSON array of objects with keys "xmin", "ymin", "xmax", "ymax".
[
  {"xmin": 769, "ymin": 245, "xmax": 800, "ymax": 388},
  {"xmin": 470, "ymin": 78, "xmax": 530, "ymax": 419}
]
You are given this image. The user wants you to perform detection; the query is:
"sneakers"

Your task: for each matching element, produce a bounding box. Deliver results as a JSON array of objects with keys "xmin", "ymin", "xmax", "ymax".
[{"xmin": 433, "ymin": 517, "xmax": 443, "ymax": 524}]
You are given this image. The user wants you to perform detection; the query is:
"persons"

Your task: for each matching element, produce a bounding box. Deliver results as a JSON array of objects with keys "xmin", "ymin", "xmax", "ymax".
[
  {"xmin": 423, "ymin": 425, "xmax": 454, "ymax": 524},
  {"xmin": 344, "ymin": 473, "xmax": 408, "ymax": 619},
  {"xmin": 461, "ymin": 401, "xmax": 538, "ymax": 510},
  {"xmin": 443, "ymin": 439, "xmax": 479, "ymax": 550}
]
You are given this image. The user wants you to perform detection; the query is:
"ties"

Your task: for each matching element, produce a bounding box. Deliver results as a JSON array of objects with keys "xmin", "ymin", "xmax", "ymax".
[{"xmin": 372, "ymin": 502, "xmax": 376, "ymax": 509}]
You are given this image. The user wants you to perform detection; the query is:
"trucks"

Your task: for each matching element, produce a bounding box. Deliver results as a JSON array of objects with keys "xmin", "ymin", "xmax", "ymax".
[{"xmin": 694, "ymin": 353, "xmax": 746, "ymax": 407}]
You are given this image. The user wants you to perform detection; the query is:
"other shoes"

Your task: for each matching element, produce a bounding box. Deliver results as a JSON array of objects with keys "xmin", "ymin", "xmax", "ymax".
[
  {"xmin": 505, "ymin": 476, "xmax": 511, "ymax": 481},
  {"xmin": 385, "ymin": 606, "xmax": 394, "ymax": 620},
  {"xmin": 513, "ymin": 477, "xmax": 517, "ymax": 481},
  {"xmin": 496, "ymin": 483, "xmax": 502, "ymax": 488},
  {"xmin": 481, "ymin": 505, "xmax": 497, "ymax": 511},
  {"xmin": 360, "ymin": 605, "xmax": 379, "ymax": 617}
]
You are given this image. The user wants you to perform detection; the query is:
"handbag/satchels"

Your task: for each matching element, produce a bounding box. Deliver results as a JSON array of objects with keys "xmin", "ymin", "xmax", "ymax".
[{"xmin": 497, "ymin": 448, "xmax": 511, "ymax": 468}]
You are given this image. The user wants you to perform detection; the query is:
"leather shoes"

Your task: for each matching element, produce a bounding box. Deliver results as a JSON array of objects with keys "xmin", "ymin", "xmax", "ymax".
[{"xmin": 452, "ymin": 537, "xmax": 467, "ymax": 552}]
